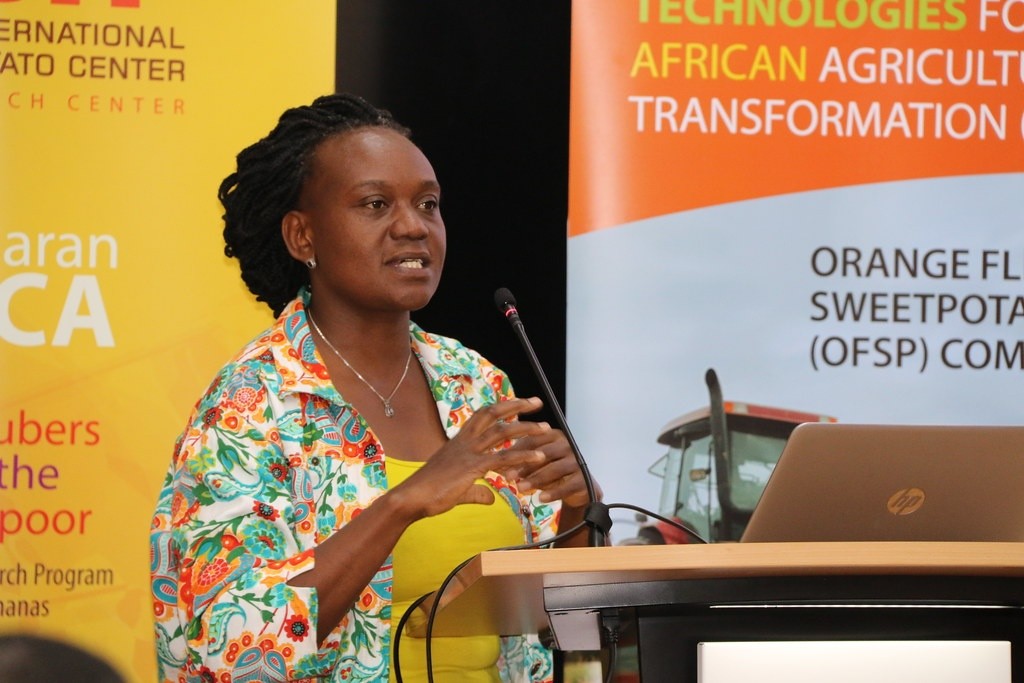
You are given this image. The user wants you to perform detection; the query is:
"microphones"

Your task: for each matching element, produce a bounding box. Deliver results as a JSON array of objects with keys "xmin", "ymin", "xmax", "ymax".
[{"xmin": 495, "ymin": 288, "xmax": 607, "ymax": 547}]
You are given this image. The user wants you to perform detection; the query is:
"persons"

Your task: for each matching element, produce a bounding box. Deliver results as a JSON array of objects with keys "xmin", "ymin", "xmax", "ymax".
[
  {"xmin": 149, "ymin": 91, "xmax": 609, "ymax": 683},
  {"xmin": 0, "ymin": 632, "xmax": 127, "ymax": 683}
]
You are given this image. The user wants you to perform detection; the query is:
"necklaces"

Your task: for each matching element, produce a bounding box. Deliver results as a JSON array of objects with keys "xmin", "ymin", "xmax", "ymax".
[{"xmin": 306, "ymin": 306, "xmax": 413, "ymax": 417}]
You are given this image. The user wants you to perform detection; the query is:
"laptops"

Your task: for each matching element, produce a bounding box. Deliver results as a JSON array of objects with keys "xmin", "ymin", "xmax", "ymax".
[{"xmin": 739, "ymin": 421, "xmax": 1024, "ymax": 544}]
